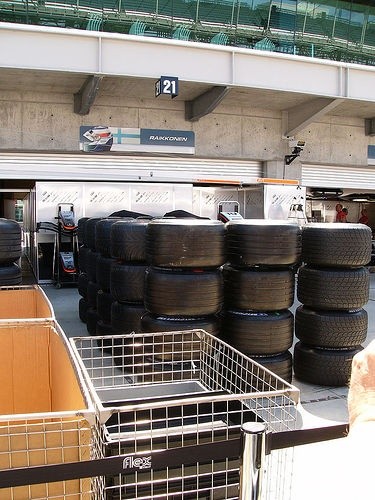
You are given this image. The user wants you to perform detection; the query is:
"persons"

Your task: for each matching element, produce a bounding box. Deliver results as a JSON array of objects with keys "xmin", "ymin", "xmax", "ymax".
[
  {"xmin": 336, "ymin": 203, "xmax": 346, "ymax": 223},
  {"xmin": 342, "ymin": 207, "xmax": 350, "ymax": 216},
  {"xmin": 356, "ymin": 207, "xmax": 370, "ymax": 225}
]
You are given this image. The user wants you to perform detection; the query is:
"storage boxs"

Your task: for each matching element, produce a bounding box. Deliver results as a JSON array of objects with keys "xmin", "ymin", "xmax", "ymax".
[
  {"xmin": 0, "ymin": 288, "xmax": 52, "ymax": 326},
  {"xmin": 0, "ymin": 323, "xmax": 92, "ymax": 499},
  {"xmin": 95, "ymin": 379, "xmax": 265, "ymax": 500}
]
